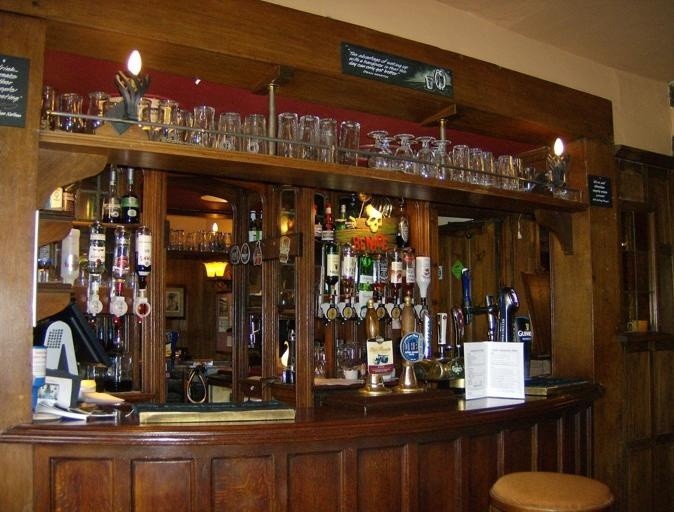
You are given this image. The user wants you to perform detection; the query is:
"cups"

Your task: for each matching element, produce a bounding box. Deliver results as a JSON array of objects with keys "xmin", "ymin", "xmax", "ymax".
[
  {"xmin": 454, "ymin": 143, "xmax": 535, "ymax": 191},
  {"xmin": 40, "ymin": 87, "xmax": 360, "ymax": 166}
]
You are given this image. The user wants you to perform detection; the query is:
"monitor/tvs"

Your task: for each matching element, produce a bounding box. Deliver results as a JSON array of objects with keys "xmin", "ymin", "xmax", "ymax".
[{"xmin": 33, "ymin": 302, "xmax": 103, "ymax": 369}]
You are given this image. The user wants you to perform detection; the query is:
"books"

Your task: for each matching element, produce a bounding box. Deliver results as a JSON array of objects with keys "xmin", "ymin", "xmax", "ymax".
[
  {"xmin": 134, "ymin": 401, "xmax": 297, "ymax": 425},
  {"xmin": 462, "ymin": 337, "xmax": 526, "ymax": 402},
  {"xmin": 31, "ymin": 370, "xmax": 127, "ymax": 427},
  {"xmin": 524, "ymin": 377, "xmax": 594, "ymax": 397}
]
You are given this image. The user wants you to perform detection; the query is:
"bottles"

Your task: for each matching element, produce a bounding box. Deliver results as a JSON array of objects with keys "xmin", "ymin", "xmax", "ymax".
[
  {"xmin": 248, "ymin": 208, "xmax": 262, "ymax": 242},
  {"xmin": 396, "ymin": 202, "xmax": 411, "ymax": 248},
  {"xmin": 313, "ymin": 203, "xmax": 350, "ymax": 240},
  {"xmin": 82, "ymin": 224, "xmax": 152, "ymax": 318},
  {"xmin": 320, "ymin": 239, "xmax": 419, "ymax": 304},
  {"xmin": 102, "ymin": 163, "xmax": 139, "ymax": 224}
]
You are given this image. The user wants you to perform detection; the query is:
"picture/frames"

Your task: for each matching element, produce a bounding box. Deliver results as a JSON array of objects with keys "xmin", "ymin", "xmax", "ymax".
[{"xmin": 166, "ymin": 282, "xmax": 185, "ymax": 318}]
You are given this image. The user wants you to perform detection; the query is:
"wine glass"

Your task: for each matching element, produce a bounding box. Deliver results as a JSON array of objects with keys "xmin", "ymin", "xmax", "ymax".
[{"xmin": 368, "ymin": 128, "xmax": 452, "ymax": 180}]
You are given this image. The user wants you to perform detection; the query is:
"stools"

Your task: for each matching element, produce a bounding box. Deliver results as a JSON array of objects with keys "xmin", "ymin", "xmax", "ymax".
[{"xmin": 486, "ymin": 472, "xmax": 615, "ymax": 512}]
